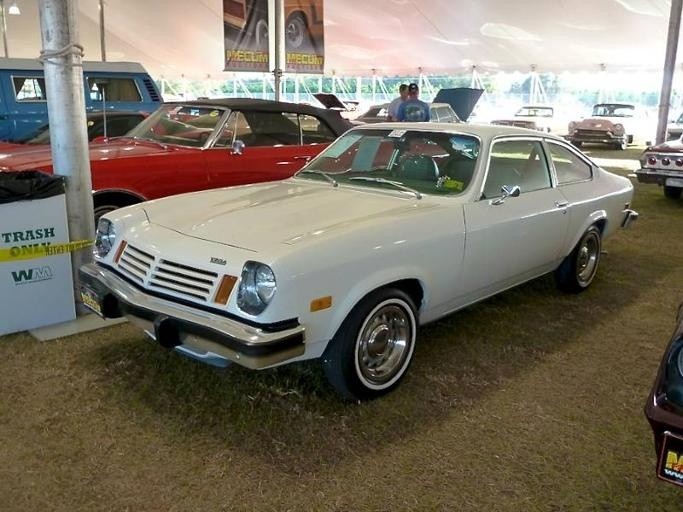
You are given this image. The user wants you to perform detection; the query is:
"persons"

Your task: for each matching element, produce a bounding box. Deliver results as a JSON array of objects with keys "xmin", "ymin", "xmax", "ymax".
[
  {"xmin": 396, "ymin": 83, "xmax": 431, "ymax": 121},
  {"xmin": 384, "ymin": 82, "xmax": 409, "ymax": 121}
]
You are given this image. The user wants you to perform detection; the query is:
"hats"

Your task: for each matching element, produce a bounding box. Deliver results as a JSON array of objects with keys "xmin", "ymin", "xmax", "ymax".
[{"xmin": 409, "ymin": 83, "xmax": 417, "ymax": 91}]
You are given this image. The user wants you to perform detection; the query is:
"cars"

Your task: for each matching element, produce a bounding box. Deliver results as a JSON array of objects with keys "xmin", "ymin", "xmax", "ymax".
[
  {"xmin": 24, "ymin": 111, "xmax": 233, "ymax": 144},
  {"xmin": 76, "ymin": 119, "xmax": 639, "ymax": 400},
  {"xmin": 489, "ymin": 101, "xmax": 683, "ymax": 198}
]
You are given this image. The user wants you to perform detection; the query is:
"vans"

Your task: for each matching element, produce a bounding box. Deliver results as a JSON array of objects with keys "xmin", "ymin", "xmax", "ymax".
[{"xmin": 0, "ymin": 57, "xmax": 164, "ymax": 142}]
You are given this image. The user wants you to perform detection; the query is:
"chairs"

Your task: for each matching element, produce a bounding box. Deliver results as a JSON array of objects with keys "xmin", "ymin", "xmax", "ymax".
[{"xmin": 397, "ymin": 151, "xmax": 477, "ymax": 184}]
